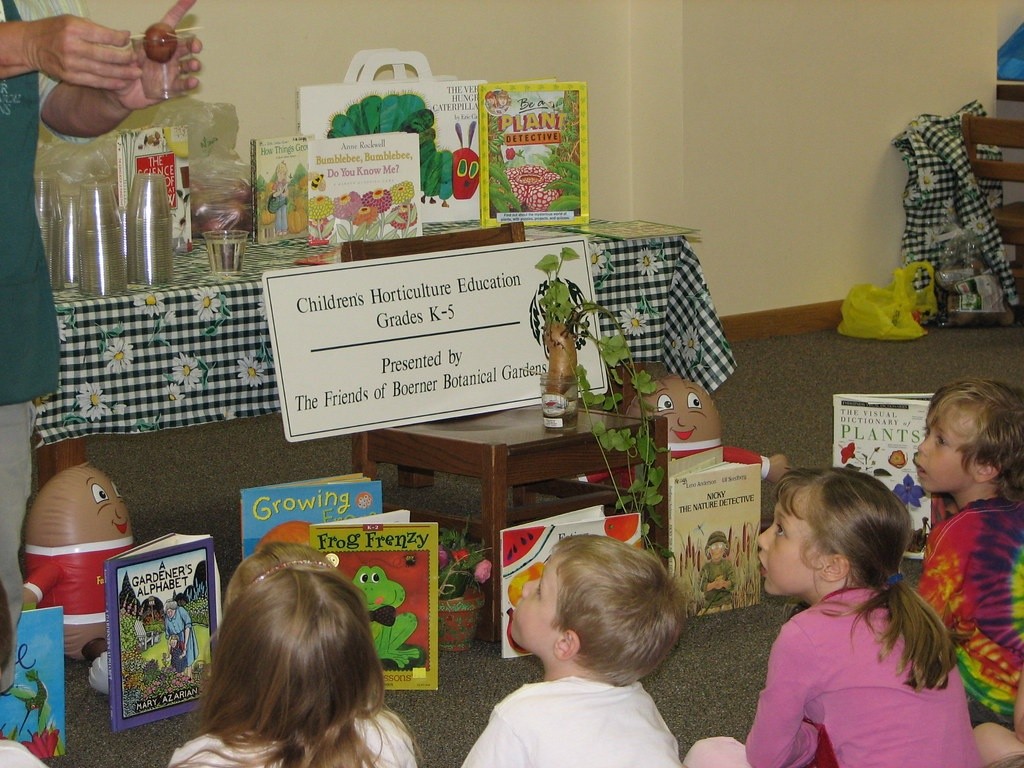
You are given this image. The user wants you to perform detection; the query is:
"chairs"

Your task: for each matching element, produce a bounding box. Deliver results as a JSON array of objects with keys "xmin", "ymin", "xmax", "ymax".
[{"xmin": 954, "ymin": 81, "xmax": 1024, "ymax": 300}]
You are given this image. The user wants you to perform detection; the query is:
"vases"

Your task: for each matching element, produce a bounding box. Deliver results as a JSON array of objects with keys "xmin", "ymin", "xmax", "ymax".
[{"xmin": 438, "ymin": 594, "xmax": 484, "ymax": 650}]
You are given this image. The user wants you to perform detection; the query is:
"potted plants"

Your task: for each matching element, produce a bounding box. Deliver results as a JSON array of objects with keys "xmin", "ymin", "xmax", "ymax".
[{"xmin": 535, "ymin": 248, "xmax": 673, "ymax": 580}]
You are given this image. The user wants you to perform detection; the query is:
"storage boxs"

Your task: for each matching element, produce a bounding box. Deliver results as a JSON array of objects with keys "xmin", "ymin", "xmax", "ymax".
[{"xmin": 294, "ymin": 47, "xmax": 489, "ymax": 224}]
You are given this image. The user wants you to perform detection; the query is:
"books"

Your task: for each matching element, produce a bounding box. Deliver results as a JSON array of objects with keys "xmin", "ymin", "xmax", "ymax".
[
  {"xmin": 561, "ymin": 219, "xmax": 701, "ymax": 240},
  {"xmin": 250, "ymin": 130, "xmax": 423, "ymax": 246},
  {"xmin": 240, "ymin": 473, "xmax": 438, "ymax": 690},
  {"xmin": 477, "ymin": 77, "xmax": 589, "ymax": 225},
  {"xmin": 117, "ymin": 125, "xmax": 193, "ymax": 252},
  {"xmin": 500, "ymin": 504, "xmax": 642, "ymax": 659},
  {"xmin": 105, "ymin": 533, "xmax": 218, "ymax": 734},
  {"xmin": 293, "ymin": 248, "xmax": 342, "ymax": 264},
  {"xmin": 831, "ymin": 392, "xmax": 936, "ymax": 560},
  {"xmin": 669, "ymin": 458, "xmax": 762, "ymax": 616}
]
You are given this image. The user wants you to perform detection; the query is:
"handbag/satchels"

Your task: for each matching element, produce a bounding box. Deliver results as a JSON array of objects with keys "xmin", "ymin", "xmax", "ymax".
[
  {"xmin": 837, "ymin": 262, "xmax": 938, "ymax": 340},
  {"xmin": 296, "ymin": 46, "xmax": 487, "ymax": 223}
]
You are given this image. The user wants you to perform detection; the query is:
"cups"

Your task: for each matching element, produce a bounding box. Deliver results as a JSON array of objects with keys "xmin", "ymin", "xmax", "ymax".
[
  {"xmin": 131, "ymin": 32, "xmax": 194, "ymax": 99},
  {"xmin": 204, "ymin": 230, "xmax": 249, "ymax": 275},
  {"xmin": 35, "ymin": 173, "xmax": 171, "ymax": 298}
]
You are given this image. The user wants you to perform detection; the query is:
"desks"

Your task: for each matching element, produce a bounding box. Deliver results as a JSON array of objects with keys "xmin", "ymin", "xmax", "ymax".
[
  {"xmin": 350, "ymin": 406, "xmax": 673, "ymax": 645},
  {"xmin": 36, "ymin": 220, "xmax": 737, "ymax": 487}
]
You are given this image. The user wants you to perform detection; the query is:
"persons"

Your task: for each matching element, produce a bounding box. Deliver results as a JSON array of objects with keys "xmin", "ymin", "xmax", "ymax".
[
  {"xmin": 0, "ymin": 0, "xmax": 205, "ymax": 686},
  {"xmin": 168, "ymin": 540, "xmax": 425, "ymax": 768},
  {"xmin": 914, "ymin": 377, "xmax": 1024, "ymax": 733},
  {"xmin": 460, "ymin": 533, "xmax": 694, "ymax": 768},
  {"xmin": 0, "ymin": 579, "xmax": 54, "ymax": 768},
  {"xmin": 684, "ymin": 465, "xmax": 1024, "ymax": 768}
]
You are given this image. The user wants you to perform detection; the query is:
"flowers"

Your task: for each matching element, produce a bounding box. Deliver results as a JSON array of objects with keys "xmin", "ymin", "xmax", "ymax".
[{"xmin": 438, "ymin": 525, "xmax": 494, "ymax": 597}]
